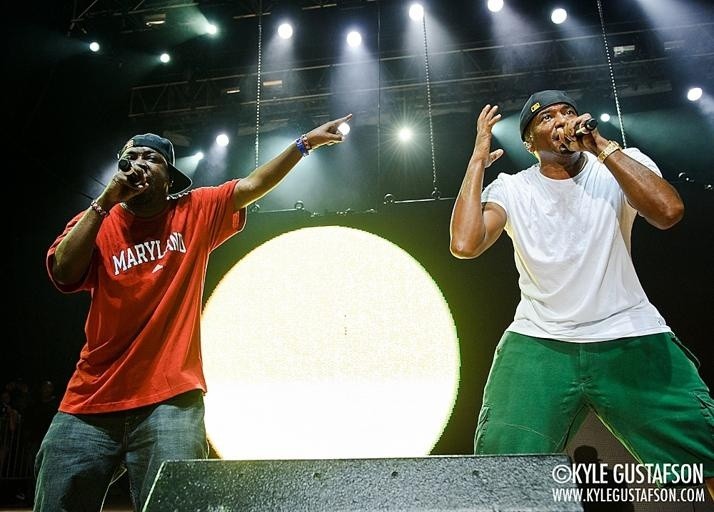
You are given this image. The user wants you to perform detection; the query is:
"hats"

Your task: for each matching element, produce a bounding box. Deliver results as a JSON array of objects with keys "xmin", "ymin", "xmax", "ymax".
[
  {"xmin": 118, "ymin": 132, "xmax": 193, "ymax": 196},
  {"xmin": 519, "ymin": 89, "xmax": 579, "ymax": 141},
  {"xmin": 574, "ymin": 445, "xmax": 604, "ymax": 463}
]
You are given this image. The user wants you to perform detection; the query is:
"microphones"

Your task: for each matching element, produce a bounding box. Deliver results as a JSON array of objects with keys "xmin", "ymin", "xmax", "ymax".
[
  {"xmin": 117, "ymin": 159, "xmax": 145, "ymax": 185},
  {"xmin": 551, "ymin": 116, "xmax": 597, "ymax": 151}
]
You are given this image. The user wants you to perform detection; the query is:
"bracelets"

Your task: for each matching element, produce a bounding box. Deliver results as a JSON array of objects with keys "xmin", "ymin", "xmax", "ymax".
[
  {"xmin": 598, "ymin": 141, "xmax": 620, "ymax": 163},
  {"xmin": 301, "ymin": 133, "xmax": 312, "ymax": 150},
  {"xmin": 90, "ymin": 201, "xmax": 110, "ymax": 217},
  {"xmin": 296, "ymin": 137, "xmax": 309, "ymax": 157}
]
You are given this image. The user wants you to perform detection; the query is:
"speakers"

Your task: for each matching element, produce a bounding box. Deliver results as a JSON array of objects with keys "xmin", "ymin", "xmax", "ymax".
[
  {"xmin": 142, "ymin": 453, "xmax": 584, "ymax": 512},
  {"xmin": 569, "ymin": 402, "xmax": 714, "ymax": 512}
]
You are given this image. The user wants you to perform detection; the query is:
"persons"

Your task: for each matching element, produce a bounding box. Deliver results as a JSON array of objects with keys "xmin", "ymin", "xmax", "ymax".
[
  {"xmin": 572, "ymin": 443, "xmax": 636, "ymax": 511},
  {"xmin": 0, "ymin": 377, "xmax": 57, "ymax": 500},
  {"xmin": 451, "ymin": 89, "xmax": 713, "ymax": 511},
  {"xmin": 31, "ymin": 112, "xmax": 356, "ymax": 512}
]
